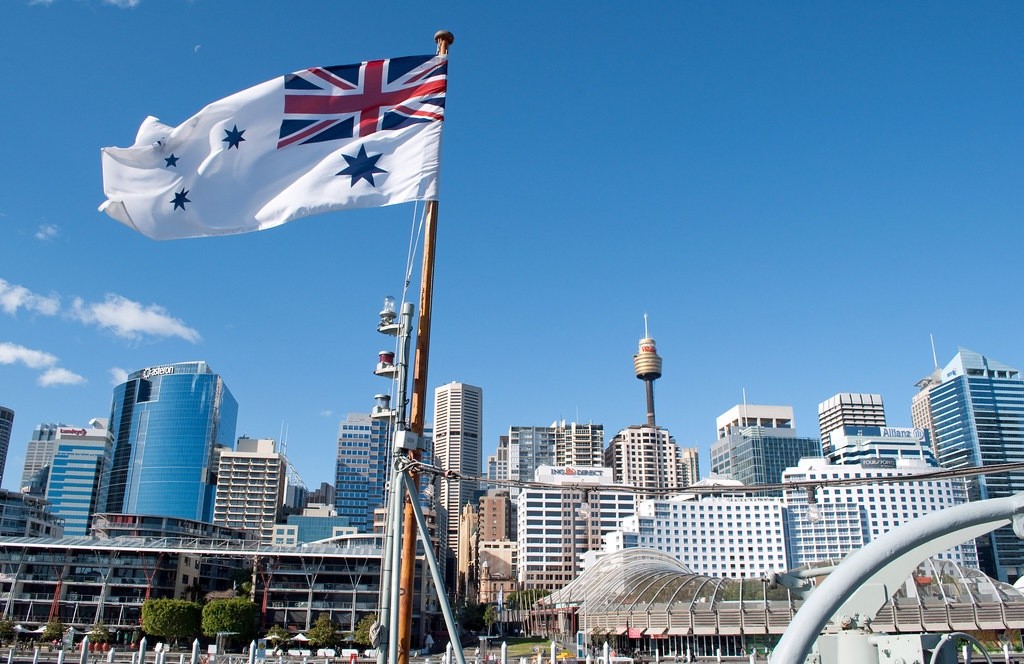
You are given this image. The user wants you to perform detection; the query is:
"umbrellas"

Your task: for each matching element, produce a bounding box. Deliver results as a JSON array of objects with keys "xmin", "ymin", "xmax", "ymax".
[
  {"xmin": 290, "ymin": 632, "xmax": 312, "ymax": 649},
  {"xmin": 13, "ymin": 624, "xmax": 30, "ymax": 640},
  {"xmin": 263, "ymin": 633, "xmax": 288, "ymax": 646},
  {"xmin": 341, "ymin": 634, "xmax": 356, "ymax": 649},
  {"xmin": 30, "ymin": 626, "xmax": 60, "ymax": 642},
  {"xmin": 83, "ymin": 628, "xmax": 104, "ymax": 634},
  {"xmin": 62, "ymin": 627, "xmax": 82, "ymax": 634}
]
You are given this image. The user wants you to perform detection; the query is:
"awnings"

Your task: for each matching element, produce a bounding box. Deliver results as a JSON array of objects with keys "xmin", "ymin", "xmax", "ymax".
[
  {"xmin": 667, "ymin": 628, "xmax": 690, "ymax": 635},
  {"xmin": 627, "ymin": 628, "xmax": 647, "ymax": 638},
  {"xmin": 719, "ymin": 628, "xmax": 740, "ymax": 635},
  {"xmin": 744, "ymin": 627, "xmax": 766, "ymax": 634},
  {"xmin": 644, "ymin": 628, "xmax": 667, "ymax": 636},
  {"xmin": 583, "ymin": 628, "xmax": 594, "ymax": 634},
  {"xmin": 591, "ymin": 645, "xmax": 594, "ymax": 659},
  {"xmin": 590, "ymin": 628, "xmax": 602, "ymax": 635},
  {"xmin": 599, "ymin": 627, "xmax": 614, "ymax": 635},
  {"xmin": 610, "ymin": 628, "xmax": 627, "ymax": 635},
  {"xmin": 769, "ymin": 627, "xmax": 788, "ymax": 634},
  {"xmin": 694, "ymin": 628, "xmax": 716, "ymax": 635}
]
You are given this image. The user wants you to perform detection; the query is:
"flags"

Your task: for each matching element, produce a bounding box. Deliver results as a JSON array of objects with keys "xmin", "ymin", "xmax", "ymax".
[{"xmin": 97, "ymin": 53, "xmax": 447, "ymax": 240}]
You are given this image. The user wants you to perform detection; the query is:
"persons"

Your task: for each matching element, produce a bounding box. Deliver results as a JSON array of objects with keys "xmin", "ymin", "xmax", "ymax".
[
  {"xmin": 741, "ymin": 646, "xmax": 759, "ymax": 657},
  {"xmin": 620, "ymin": 647, "xmax": 634, "ymax": 658},
  {"xmin": 335, "ymin": 645, "xmax": 342, "ymax": 657},
  {"xmin": 675, "ymin": 653, "xmax": 695, "ymax": 663}
]
